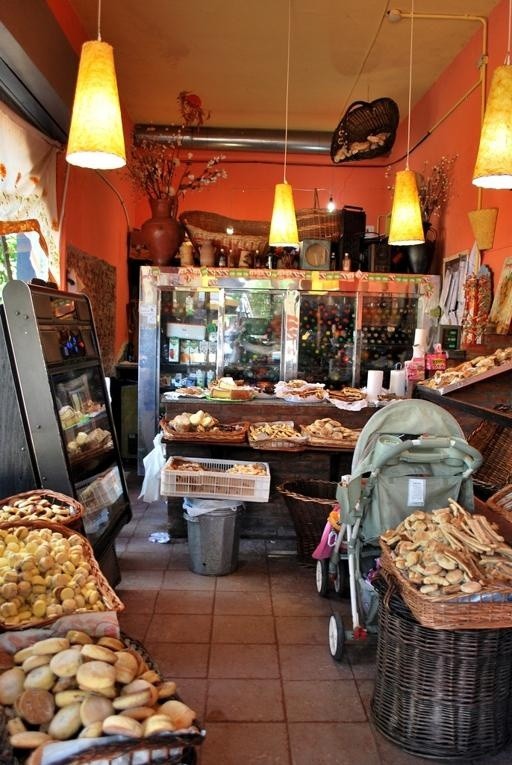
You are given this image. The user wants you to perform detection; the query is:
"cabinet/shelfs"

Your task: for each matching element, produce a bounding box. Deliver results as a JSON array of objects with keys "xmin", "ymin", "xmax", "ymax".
[
  {"xmin": 159, "ymin": 393, "xmax": 385, "ymax": 540},
  {"xmin": 0, "ymin": 279, "xmax": 133, "ymax": 589}
]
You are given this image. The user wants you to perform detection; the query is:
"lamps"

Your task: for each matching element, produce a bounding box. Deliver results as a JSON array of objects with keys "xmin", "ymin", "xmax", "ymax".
[
  {"xmin": 385, "ymin": 0, "xmax": 426, "ymax": 246},
  {"xmin": 64, "ymin": 0, "xmax": 127, "ymax": 170},
  {"xmin": 325, "ymin": 191, "xmax": 335, "ymax": 211},
  {"xmin": 470, "ymin": 0, "xmax": 512, "ymax": 190}
]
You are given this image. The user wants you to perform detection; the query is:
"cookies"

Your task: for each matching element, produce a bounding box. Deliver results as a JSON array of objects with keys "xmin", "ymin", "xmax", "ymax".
[{"xmin": 0, "ymin": 495, "xmax": 196, "ymax": 765}]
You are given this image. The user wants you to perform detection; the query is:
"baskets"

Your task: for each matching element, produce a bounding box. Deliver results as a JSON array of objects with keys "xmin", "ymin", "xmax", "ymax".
[
  {"xmin": 159, "ymin": 456, "xmax": 271, "ymax": 503},
  {"xmin": 280, "ymin": 479, "xmax": 338, "ymax": 567},
  {"xmin": 377, "ymin": 535, "xmax": 512, "ymax": 630},
  {"xmin": 159, "ymin": 418, "xmax": 511, "ymax": 760},
  {"xmin": 159, "ymin": 418, "xmax": 361, "ymax": 451},
  {"xmin": 330, "ymin": 97, "xmax": 399, "ymax": 163},
  {"xmin": 179, "ymin": 188, "xmax": 343, "ymax": 257},
  {"xmin": 369, "ymin": 573, "xmax": 512, "ymax": 763},
  {"xmin": 0, "ymin": 466, "xmax": 207, "ymax": 765},
  {"xmin": 0, "ymin": 466, "xmax": 207, "ymax": 764}
]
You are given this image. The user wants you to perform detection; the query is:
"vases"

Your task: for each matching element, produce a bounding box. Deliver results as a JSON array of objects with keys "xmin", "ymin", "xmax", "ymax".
[
  {"xmin": 400, "ymin": 220, "xmax": 435, "ymax": 274},
  {"xmin": 140, "ymin": 197, "xmax": 182, "ymax": 265}
]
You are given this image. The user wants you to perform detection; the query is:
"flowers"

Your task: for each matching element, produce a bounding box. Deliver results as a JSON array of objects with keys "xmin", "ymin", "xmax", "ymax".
[
  {"xmin": 126, "ymin": 121, "xmax": 229, "ymax": 199},
  {"xmin": 385, "ymin": 150, "xmax": 460, "ymax": 221}
]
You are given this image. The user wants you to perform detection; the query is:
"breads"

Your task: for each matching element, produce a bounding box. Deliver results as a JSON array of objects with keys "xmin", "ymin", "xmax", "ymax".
[
  {"xmin": 60, "ymin": 399, "xmax": 114, "ymax": 500},
  {"xmin": 381, "ymin": 497, "xmax": 512, "ymax": 596},
  {"xmin": 170, "ymin": 385, "xmax": 364, "ymax": 476},
  {"xmin": 427, "ymin": 347, "xmax": 512, "ymax": 389}
]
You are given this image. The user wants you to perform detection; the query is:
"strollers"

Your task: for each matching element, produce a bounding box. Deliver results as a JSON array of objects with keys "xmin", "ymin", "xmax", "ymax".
[{"xmin": 314, "ymin": 397, "xmax": 484, "ymax": 662}]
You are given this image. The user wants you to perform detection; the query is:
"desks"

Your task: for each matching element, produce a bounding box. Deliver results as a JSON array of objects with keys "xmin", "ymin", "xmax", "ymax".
[{"xmin": 267, "ymin": 0, "xmax": 299, "ymax": 247}]
